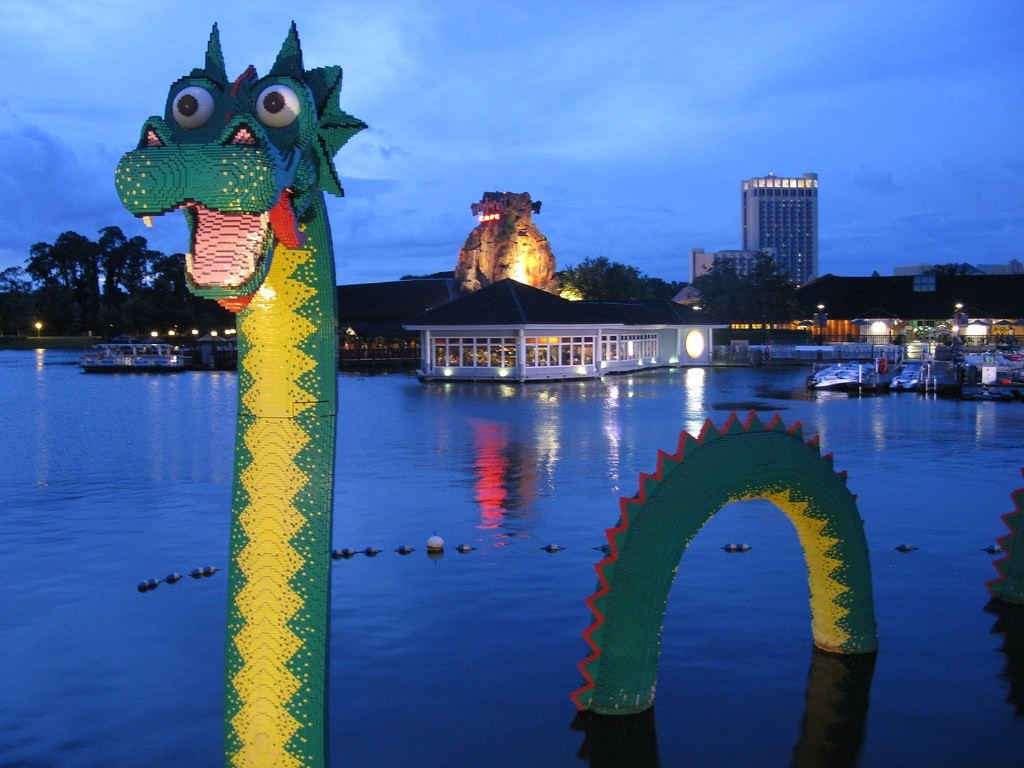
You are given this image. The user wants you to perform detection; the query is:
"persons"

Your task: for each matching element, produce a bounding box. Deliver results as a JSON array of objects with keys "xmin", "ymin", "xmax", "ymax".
[{"xmin": 996, "ymin": 327, "xmax": 1015, "ymax": 343}]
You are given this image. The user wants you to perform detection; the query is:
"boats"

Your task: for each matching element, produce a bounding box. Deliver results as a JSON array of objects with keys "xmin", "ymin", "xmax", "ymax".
[
  {"xmin": 950, "ymin": 347, "xmax": 1024, "ymax": 399},
  {"xmin": 805, "ymin": 357, "xmax": 890, "ymax": 391},
  {"xmin": 885, "ymin": 360, "xmax": 964, "ymax": 393},
  {"xmin": 78, "ymin": 342, "xmax": 193, "ymax": 373}
]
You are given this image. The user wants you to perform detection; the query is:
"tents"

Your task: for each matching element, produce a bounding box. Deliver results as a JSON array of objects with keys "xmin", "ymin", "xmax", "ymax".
[{"xmin": 968, "ymin": 319, "xmax": 1024, "ymax": 344}]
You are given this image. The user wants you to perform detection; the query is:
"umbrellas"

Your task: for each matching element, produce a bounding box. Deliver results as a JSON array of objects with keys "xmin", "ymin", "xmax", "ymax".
[
  {"xmin": 798, "ymin": 321, "xmax": 813, "ymax": 330},
  {"xmin": 846, "ymin": 319, "xmax": 871, "ymax": 340}
]
